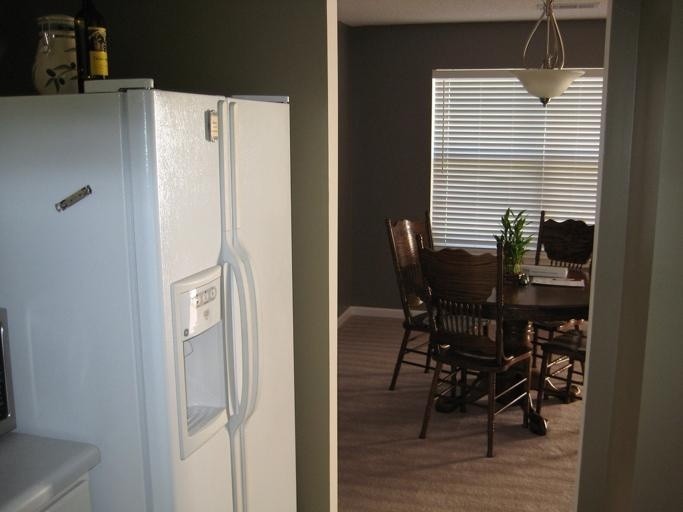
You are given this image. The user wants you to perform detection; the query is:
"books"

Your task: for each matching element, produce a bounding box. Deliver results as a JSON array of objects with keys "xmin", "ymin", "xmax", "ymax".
[{"xmin": 521, "ymin": 265, "xmax": 568, "ymax": 278}]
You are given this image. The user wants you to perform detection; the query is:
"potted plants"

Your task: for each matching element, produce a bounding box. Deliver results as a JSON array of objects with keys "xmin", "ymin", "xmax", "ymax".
[{"xmin": 494, "ymin": 208, "xmax": 535, "ymax": 288}]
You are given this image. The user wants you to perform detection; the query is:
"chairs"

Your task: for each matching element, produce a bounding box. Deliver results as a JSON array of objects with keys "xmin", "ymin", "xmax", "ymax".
[
  {"xmin": 383, "ymin": 213, "xmax": 489, "ymax": 391},
  {"xmin": 536, "ymin": 330, "xmax": 587, "ymax": 414},
  {"xmin": 531, "ymin": 209, "xmax": 595, "ymax": 367},
  {"xmin": 416, "ymin": 232, "xmax": 535, "ymax": 457}
]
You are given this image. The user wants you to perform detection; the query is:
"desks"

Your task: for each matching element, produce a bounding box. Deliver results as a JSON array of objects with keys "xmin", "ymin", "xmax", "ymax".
[{"xmin": 415, "ymin": 271, "xmax": 590, "ymax": 435}]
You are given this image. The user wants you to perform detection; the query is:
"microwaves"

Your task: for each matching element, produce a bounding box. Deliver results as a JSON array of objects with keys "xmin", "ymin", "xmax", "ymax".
[
  {"xmin": 0, "ymin": 308, "xmax": 18, "ymax": 436},
  {"xmin": 0, "ymin": 93, "xmax": 299, "ymax": 508}
]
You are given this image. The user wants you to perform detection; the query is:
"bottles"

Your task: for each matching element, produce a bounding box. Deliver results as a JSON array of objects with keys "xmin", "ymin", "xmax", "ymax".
[{"xmin": 37, "ymin": 14, "xmax": 109, "ymax": 92}]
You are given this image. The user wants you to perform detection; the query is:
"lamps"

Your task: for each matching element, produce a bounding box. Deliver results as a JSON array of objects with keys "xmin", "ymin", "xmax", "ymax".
[{"xmin": 508, "ymin": 0, "xmax": 586, "ymax": 108}]
